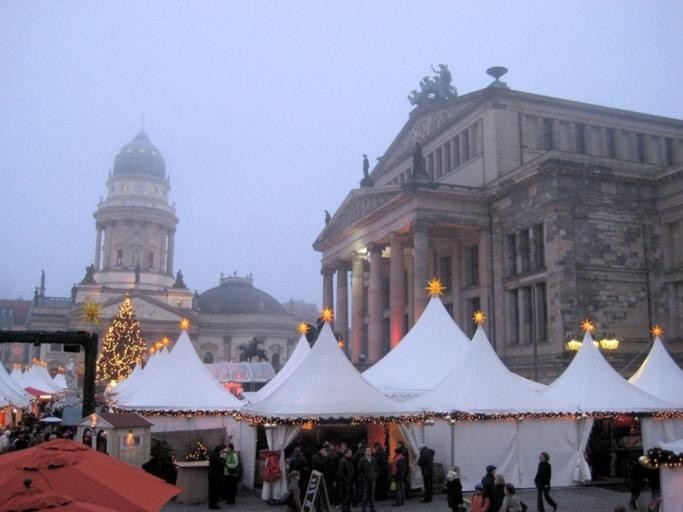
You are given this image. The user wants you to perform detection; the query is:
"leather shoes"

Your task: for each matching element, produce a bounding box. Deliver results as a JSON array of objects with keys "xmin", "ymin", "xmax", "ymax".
[{"xmin": 553, "ymin": 504, "xmax": 556, "ymax": 511}]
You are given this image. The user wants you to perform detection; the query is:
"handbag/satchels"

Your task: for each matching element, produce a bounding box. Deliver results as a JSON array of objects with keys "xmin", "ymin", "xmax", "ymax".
[
  {"xmin": 519, "ymin": 502, "xmax": 527, "ymax": 512},
  {"xmin": 227, "ymin": 454, "xmax": 240, "ymax": 474}
]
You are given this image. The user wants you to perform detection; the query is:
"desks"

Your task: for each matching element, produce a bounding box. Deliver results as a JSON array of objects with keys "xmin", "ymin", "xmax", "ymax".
[{"xmin": 176, "ymin": 461, "xmax": 210, "ymax": 504}]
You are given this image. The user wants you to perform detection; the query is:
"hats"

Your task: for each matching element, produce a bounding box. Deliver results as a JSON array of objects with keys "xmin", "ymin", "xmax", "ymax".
[
  {"xmin": 486, "ymin": 466, "xmax": 495, "ymax": 472},
  {"xmin": 474, "ymin": 484, "xmax": 484, "ymax": 491}
]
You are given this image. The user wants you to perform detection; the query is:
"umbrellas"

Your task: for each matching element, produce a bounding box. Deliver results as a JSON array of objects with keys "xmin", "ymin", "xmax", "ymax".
[
  {"xmin": 0, "ymin": 437, "xmax": 185, "ymax": 512},
  {"xmin": 0, "ymin": 477, "xmax": 122, "ymax": 512}
]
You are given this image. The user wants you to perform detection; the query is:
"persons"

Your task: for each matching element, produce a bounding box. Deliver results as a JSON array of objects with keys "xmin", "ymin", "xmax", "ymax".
[
  {"xmin": 275, "ymin": 435, "xmax": 412, "ymax": 512},
  {"xmin": 221, "ymin": 443, "xmax": 240, "ymax": 506},
  {"xmin": 533, "ymin": 451, "xmax": 558, "ymax": 511},
  {"xmin": 416, "ymin": 444, "xmax": 436, "ymax": 504},
  {"xmin": 480, "ymin": 463, "xmax": 498, "ymax": 511},
  {"xmin": 613, "ymin": 488, "xmax": 660, "ymax": 511},
  {"xmin": 493, "ymin": 473, "xmax": 505, "ymax": 507},
  {"xmin": 205, "ymin": 444, "xmax": 227, "ymax": 510},
  {"xmin": 498, "ymin": 482, "xmax": 523, "ymax": 511},
  {"xmin": 468, "ymin": 482, "xmax": 490, "ymax": 511},
  {"xmin": 0, "ymin": 392, "xmax": 178, "ymax": 486},
  {"xmin": 445, "ymin": 464, "xmax": 466, "ymax": 512}
]
